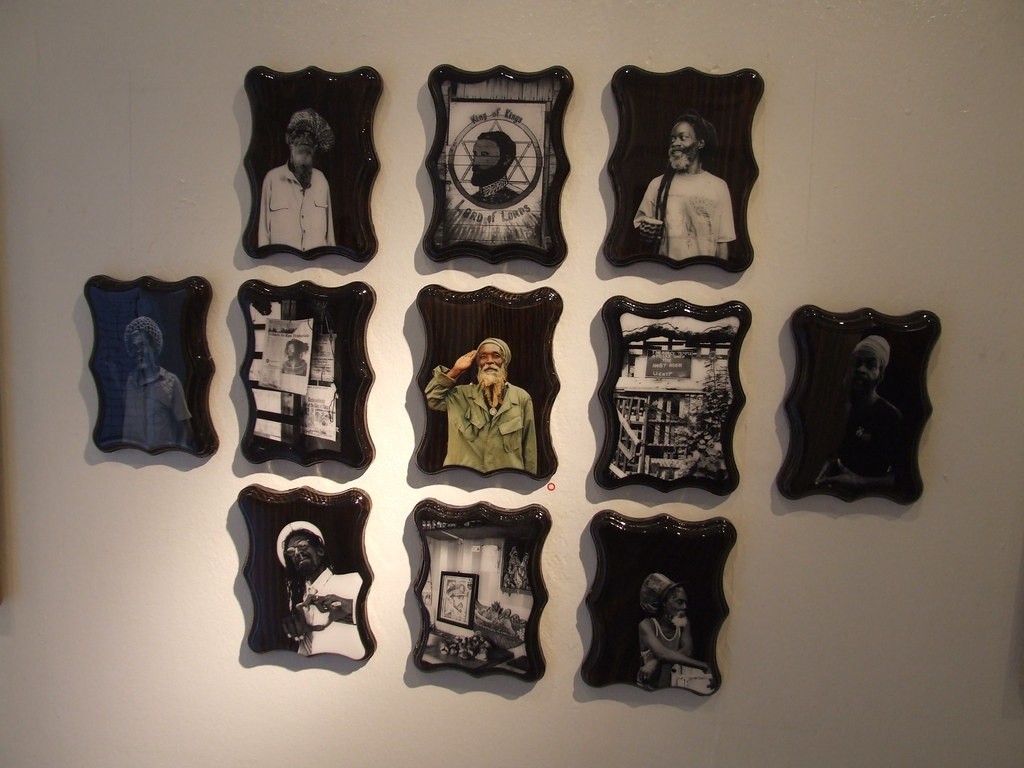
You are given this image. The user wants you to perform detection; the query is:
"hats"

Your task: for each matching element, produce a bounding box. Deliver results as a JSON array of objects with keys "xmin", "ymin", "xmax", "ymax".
[
  {"xmin": 277, "ymin": 520, "xmax": 327, "ymax": 567},
  {"xmin": 852, "ymin": 334, "xmax": 890, "ymax": 371},
  {"xmin": 641, "ymin": 572, "xmax": 681, "ymax": 614}
]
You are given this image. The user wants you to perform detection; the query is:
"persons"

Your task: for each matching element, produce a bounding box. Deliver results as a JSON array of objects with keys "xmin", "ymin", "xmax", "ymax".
[
  {"xmin": 637, "ymin": 573, "xmax": 711, "ymax": 689},
  {"xmin": 633, "ymin": 117, "xmax": 736, "ymax": 259},
  {"xmin": 425, "ymin": 337, "xmax": 537, "ymax": 474},
  {"xmin": 124, "ymin": 316, "xmax": 195, "ymax": 447},
  {"xmin": 258, "ymin": 112, "xmax": 336, "ymax": 252},
  {"xmin": 281, "ymin": 339, "xmax": 309, "ymax": 375},
  {"xmin": 449, "ymin": 585, "xmax": 467, "ymax": 612},
  {"xmin": 505, "ymin": 549, "xmax": 532, "ymax": 591},
  {"xmin": 827, "ymin": 334, "xmax": 901, "ymax": 502},
  {"xmin": 281, "ymin": 521, "xmax": 366, "ymax": 659}
]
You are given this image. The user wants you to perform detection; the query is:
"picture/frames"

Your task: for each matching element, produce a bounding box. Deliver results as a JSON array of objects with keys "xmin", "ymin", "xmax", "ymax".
[
  {"xmin": 82, "ymin": 275, "xmax": 219, "ymax": 459},
  {"xmin": 412, "ymin": 498, "xmax": 553, "ymax": 683},
  {"xmin": 422, "ymin": 64, "xmax": 574, "ymax": 266},
  {"xmin": 593, "ymin": 296, "xmax": 753, "ymax": 496},
  {"xmin": 237, "ymin": 483, "xmax": 377, "ymax": 661},
  {"xmin": 603, "ymin": 65, "xmax": 765, "ymax": 273},
  {"xmin": 237, "ymin": 279, "xmax": 377, "ymax": 471},
  {"xmin": 581, "ymin": 510, "xmax": 737, "ymax": 695},
  {"xmin": 416, "ymin": 284, "xmax": 563, "ymax": 481},
  {"xmin": 242, "ymin": 65, "xmax": 383, "ymax": 264},
  {"xmin": 776, "ymin": 304, "xmax": 942, "ymax": 506}
]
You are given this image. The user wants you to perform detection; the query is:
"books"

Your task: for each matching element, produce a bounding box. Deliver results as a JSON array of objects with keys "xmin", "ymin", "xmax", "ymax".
[{"xmin": 815, "ymin": 462, "xmax": 850, "ymax": 499}]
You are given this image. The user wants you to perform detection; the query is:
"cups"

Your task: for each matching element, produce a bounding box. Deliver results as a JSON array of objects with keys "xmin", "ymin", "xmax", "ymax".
[{"xmin": 639, "ymin": 217, "xmax": 663, "ymax": 245}]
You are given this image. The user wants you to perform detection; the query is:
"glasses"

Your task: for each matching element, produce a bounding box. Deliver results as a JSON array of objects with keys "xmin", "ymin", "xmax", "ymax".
[{"xmin": 286, "ymin": 537, "xmax": 310, "ymax": 556}]
